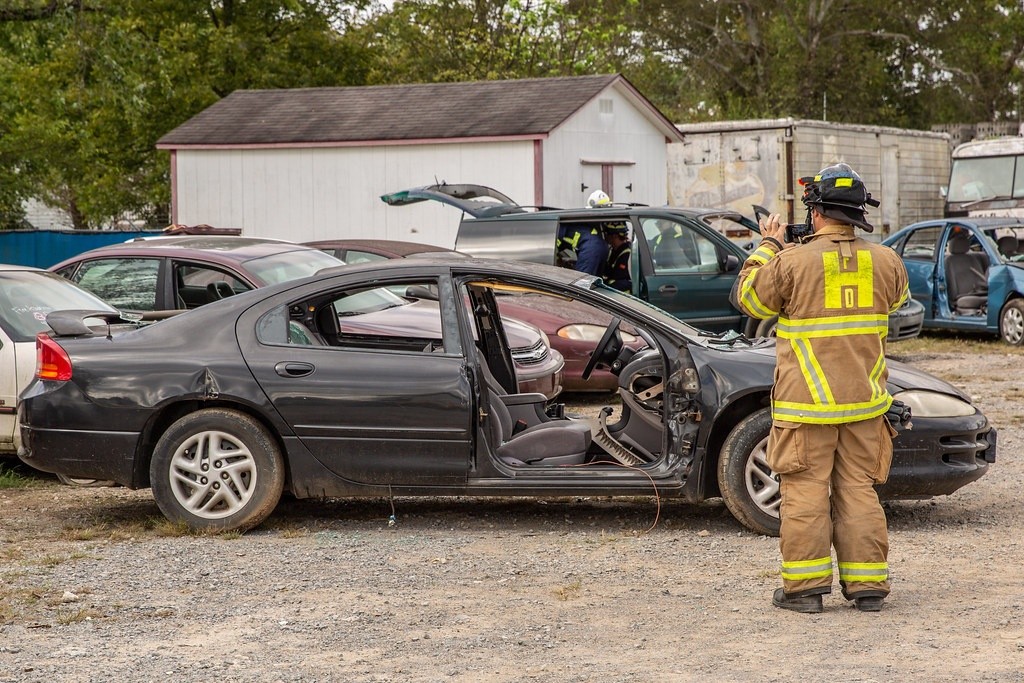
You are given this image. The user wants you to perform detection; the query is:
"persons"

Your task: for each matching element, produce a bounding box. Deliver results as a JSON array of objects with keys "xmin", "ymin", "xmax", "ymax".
[
  {"xmin": 645, "ymin": 218, "xmax": 699, "ymax": 271},
  {"xmin": 585, "ymin": 189, "xmax": 613, "ymax": 209},
  {"xmin": 556, "ymin": 221, "xmax": 608, "ymax": 277},
  {"xmin": 602, "ymin": 222, "xmax": 633, "ymax": 296},
  {"xmin": 728, "ymin": 161, "xmax": 912, "ymax": 612}
]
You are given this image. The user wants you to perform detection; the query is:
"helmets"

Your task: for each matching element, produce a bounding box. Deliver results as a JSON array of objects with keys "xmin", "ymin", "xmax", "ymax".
[
  {"xmin": 586, "ymin": 190, "xmax": 611, "ymax": 208},
  {"xmin": 602, "ymin": 221, "xmax": 628, "ymax": 236},
  {"xmin": 799, "ymin": 163, "xmax": 871, "ymax": 212}
]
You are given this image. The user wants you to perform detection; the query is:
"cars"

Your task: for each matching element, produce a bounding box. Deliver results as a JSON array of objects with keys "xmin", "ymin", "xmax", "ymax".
[
  {"xmin": 0, "ymin": 263, "xmax": 134, "ymax": 488},
  {"xmin": 294, "ymin": 241, "xmax": 653, "ymax": 390},
  {"xmin": 874, "ymin": 216, "xmax": 1024, "ymax": 347},
  {"xmin": 46, "ymin": 238, "xmax": 563, "ymax": 400},
  {"xmin": 12, "ymin": 254, "xmax": 994, "ymax": 537}
]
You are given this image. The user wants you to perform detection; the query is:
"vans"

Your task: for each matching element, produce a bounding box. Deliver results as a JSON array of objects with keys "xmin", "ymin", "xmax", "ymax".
[{"xmin": 383, "ymin": 180, "xmax": 926, "ymax": 344}]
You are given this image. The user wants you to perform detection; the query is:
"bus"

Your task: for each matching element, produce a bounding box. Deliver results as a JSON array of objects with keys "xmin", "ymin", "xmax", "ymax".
[{"xmin": 938, "ymin": 137, "xmax": 1024, "ymax": 241}]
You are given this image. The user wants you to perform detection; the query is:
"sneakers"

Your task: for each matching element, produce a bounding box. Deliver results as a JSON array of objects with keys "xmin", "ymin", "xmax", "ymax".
[
  {"xmin": 855, "ymin": 592, "xmax": 885, "ymax": 611},
  {"xmin": 771, "ymin": 588, "xmax": 823, "ymax": 612}
]
